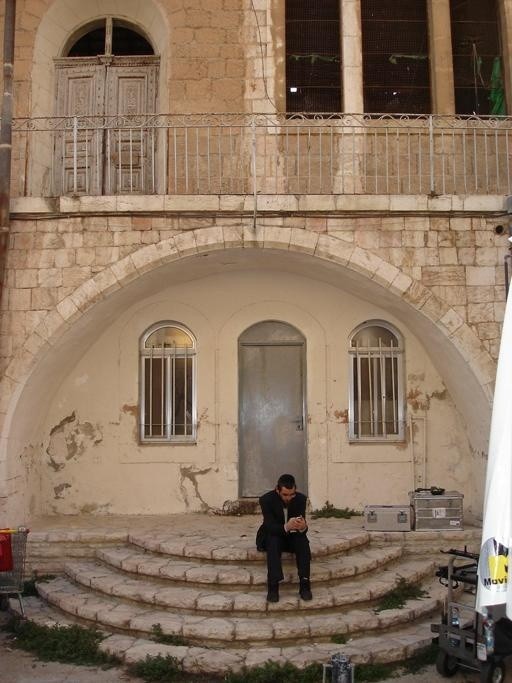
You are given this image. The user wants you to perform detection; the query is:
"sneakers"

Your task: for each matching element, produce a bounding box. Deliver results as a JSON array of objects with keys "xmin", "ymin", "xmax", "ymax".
[
  {"xmin": 267, "ymin": 584, "xmax": 279, "ymax": 603},
  {"xmin": 299, "ymin": 584, "xmax": 312, "ymax": 600}
]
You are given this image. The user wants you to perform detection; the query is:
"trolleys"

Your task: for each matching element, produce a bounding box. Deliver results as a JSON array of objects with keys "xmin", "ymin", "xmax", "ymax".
[
  {"xmin": 428, "ymin": 546, "xmax": 511, "ymax": 683},
  {"xmin": 0, "ymin": 526, "xmax": 33, "ymax": 628}
]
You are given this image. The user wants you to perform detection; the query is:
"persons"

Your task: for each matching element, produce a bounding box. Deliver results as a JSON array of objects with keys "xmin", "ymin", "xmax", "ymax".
[{"xmin": 256, "ymin": 473, "xmax": 313, "ymax": 602}]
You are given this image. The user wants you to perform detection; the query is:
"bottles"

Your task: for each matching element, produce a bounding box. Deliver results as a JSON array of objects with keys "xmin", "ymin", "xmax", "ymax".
[{"xmin": 483, "ymin": 612, "xmax": 496, "ymax": 655}]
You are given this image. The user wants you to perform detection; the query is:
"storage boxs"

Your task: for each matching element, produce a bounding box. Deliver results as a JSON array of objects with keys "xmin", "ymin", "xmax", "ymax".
[
  {"xmin": 364, "ymin": 504, "xmax": 412, "ymax": 532},
  {"xmin": 408, "ymin": 490, "xmax": 464, "ymax": 532}
]
